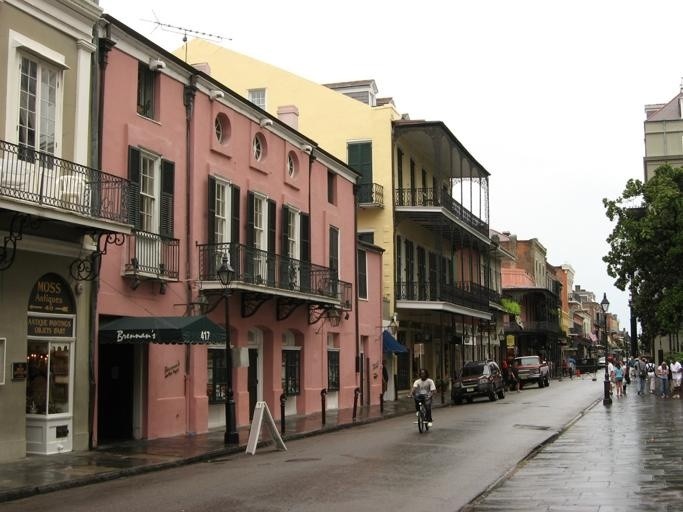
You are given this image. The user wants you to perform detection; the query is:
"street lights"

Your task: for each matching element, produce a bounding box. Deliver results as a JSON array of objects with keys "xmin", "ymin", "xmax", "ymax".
[
  {"xmin": 216, "ymin": 255, "xmax": 236, "ymax": 444},
  {"xmin": 601, "ymin": 292, "xmax": 612, "ymax": 406},
  {"xmin": 625, "ymin": 335, "xmax": 630, "ymax": 380}
]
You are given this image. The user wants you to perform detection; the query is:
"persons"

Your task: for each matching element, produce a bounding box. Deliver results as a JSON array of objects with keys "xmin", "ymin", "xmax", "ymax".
[
  {"xmin": 507, "ymin": 361, "xmax": 522, "ymax": 392},
  {"xmin": 608, "ymin": 355, "xmax": 683, "ymax": 399},
  {"xmin": 410, "ymin": 368, "xmax": 437, "ymax": 428},
  {"xmin": 381, "ymin": 359, "xmax": 388, "ymax": 403},
  {"xmin": 561, "ymin": 360, "xmax": 575, "ymax": 378}
]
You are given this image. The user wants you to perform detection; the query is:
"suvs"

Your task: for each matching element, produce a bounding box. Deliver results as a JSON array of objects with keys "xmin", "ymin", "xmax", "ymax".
[
  {"xmin": 451, "ymin": 360, "xmax": 505, "ymax": 404},
  {"xmin": 507, "ymin": 356, "xmax": 550, "ymax": 388}
]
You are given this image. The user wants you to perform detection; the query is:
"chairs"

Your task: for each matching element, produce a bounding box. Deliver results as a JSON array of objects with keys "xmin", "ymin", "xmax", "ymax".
[{"xmin": 55, "ymin": 175, "xmax": 86, "ymax": 210}]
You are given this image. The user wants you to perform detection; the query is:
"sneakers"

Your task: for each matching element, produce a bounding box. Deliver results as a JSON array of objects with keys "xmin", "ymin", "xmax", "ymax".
[
  {"xmin": 428, "ymin": 422, "xmax": 432, "ymax": 428},
  {"xmin": 672, "ymin": 395, "xmax": 680, "ymax": 399},
  {"xmin": 416, "ymin": 412, "xmax": 418, "ymax": 416}
]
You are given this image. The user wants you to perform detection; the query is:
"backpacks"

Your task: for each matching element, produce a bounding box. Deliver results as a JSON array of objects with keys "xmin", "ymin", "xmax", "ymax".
[{"xmin": 646, "ymin": 363, "xmax": 654, "ymax": 372}]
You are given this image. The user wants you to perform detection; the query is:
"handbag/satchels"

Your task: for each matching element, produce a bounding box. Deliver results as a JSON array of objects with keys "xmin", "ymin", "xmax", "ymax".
[{"xmin": 638, "ymin": 370, "xmax": 646, "ymax": 378}]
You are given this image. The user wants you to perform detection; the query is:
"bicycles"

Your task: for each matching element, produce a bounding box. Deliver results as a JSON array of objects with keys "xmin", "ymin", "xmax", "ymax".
[{"xmin": 408, "ymin": 393, "xmax": 432, "ymax": 434}]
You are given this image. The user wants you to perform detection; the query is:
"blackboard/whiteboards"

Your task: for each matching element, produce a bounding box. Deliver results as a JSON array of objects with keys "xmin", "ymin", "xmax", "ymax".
[{"xmin": 245, "ymin": 402, "xmax": 265, "ymax": 453}]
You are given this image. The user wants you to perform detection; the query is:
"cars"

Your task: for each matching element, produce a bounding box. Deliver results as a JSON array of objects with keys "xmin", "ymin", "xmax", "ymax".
[{"xmin": 597, "ymin": 357, "xmax": 606, "ymax": 369}]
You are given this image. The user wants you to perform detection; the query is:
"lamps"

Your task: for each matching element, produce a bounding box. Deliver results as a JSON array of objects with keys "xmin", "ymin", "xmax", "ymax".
[
  {"xmin": 131, "ymin": 275, "xmax": 140, "ymax": 290},
  {"xmin": 174, "ymin": 292, "xmax": 209, "ymax": 317},
  {"xmin": 315, "ymin": 309, "xmax": 340, "ymax": 335},
  {"xmin": 159, "ymin": 281, "xmax": 168, "ymax": 294},
  {"xmin": 464, "ymin": 329, "xmax": 470, "ymax": 343}
]
